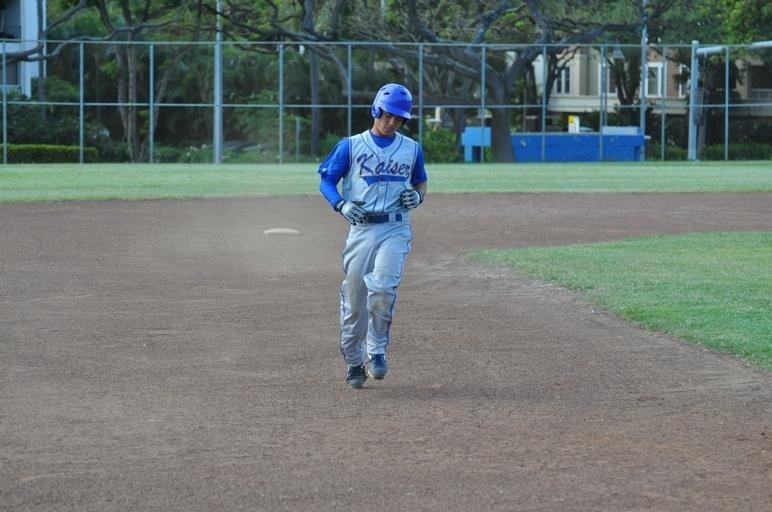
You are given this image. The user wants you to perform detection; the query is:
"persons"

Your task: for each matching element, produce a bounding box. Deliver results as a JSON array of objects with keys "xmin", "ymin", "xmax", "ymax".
[{"xmin": 317, "ymin": 81, "xmax": 427, "ymax": 390}]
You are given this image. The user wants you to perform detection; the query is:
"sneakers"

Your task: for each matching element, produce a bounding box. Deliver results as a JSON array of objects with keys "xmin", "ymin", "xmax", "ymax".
[
  {"xmin": 347, "ymin": 362, "xmax": 367, "ymax": 387},
  {"xmin": 368, "ymin": 353, "xmax": 387, "ymax": 380}
]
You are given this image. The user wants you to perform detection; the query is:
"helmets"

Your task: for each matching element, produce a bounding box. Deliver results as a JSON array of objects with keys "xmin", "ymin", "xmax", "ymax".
[{"xmin": 372, "ymin": 84, "xmax": 413, "ymax": 126}]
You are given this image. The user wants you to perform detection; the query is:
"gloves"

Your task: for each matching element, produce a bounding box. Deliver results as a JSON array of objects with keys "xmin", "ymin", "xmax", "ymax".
[
  {"xmin": 401, "ymin": 188, "xmax": 422, "ymax": 208},
  {"xmin": 335, "ymin": 199, "xmax": 367, "ymax": 225}
]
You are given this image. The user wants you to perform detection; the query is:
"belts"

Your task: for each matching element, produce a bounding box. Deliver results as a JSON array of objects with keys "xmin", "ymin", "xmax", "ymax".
[{"xmin": 367, "ymin": 214, "xmax": 402, "ymax": 224}]
